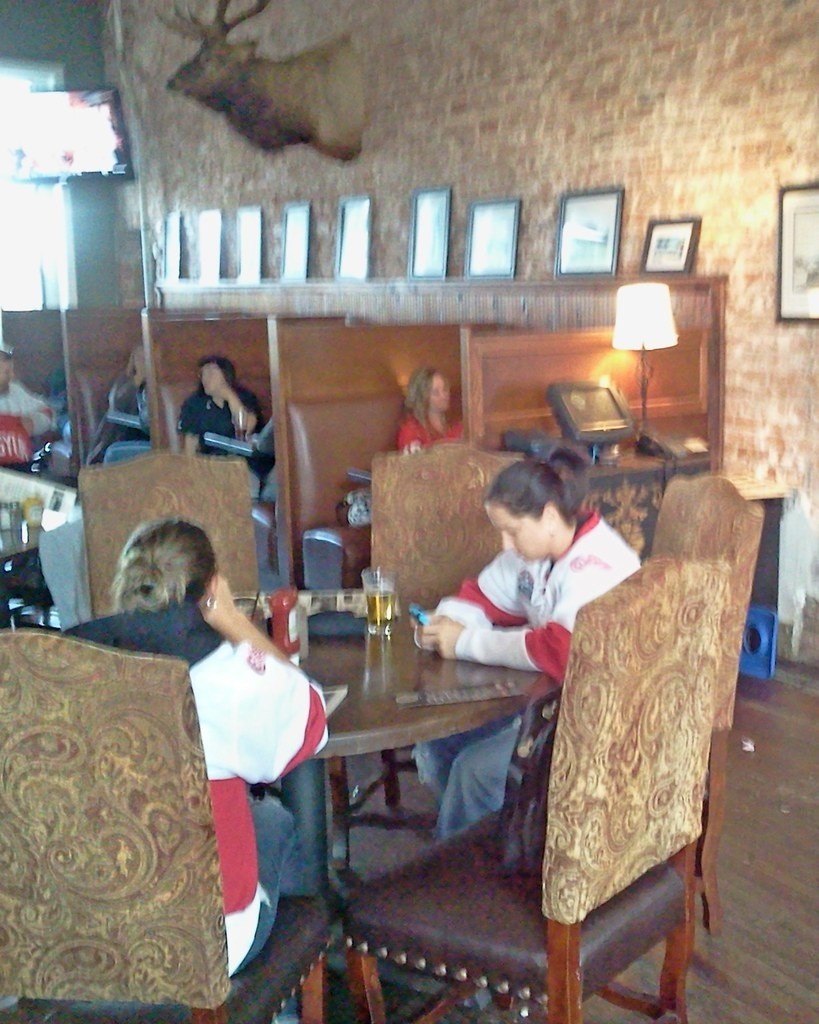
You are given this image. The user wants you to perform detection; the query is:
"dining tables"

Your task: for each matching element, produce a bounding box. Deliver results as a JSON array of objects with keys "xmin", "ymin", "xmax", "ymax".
[{"xmin": 227, "ymin": 586, "xmax": 557, "ymax": 994}]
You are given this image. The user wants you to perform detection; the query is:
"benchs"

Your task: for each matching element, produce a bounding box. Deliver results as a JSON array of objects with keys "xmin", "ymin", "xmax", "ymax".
[{"xmin": 284, "ymin": 380, "xmax": 460, "ymax": 589}]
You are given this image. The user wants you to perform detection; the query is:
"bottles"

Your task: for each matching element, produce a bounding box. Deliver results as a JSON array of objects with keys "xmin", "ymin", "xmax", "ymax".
[
  {"xmin": 0, "ymin": 503, "xmax": 10, "ymax": 531},
  {"xmin": 9, "ymin": 502, "xmax": 22, "ymax": 529},
  {"xmin": 23, "ymin": 491, "xmax": 43, "ymax": 529}
]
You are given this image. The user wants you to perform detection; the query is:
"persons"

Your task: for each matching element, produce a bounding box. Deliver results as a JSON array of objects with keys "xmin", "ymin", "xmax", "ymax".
[
  {"xmin": 58, "ymin": 517, "xmax": 330, "ymax": 1024},
  {"xmin": 176, "ymin": 354, "xmax": 267, "ymax": 503},
  {"xmin": 397, "ymin": 364, "xmax": 464, "ymax": 450},
  {"xmin": 0, "ymin": 348, "xmax": 53, "ymax": 474},
  {"xmin": 249, "ymin": 414, "xmax": 279, "ymax": 503},
  {"xmin": 86, "ymin": 344, "xmax": 151, "ymax": 463},
  {"xmin": 408, "ymin": 444, "xmax": 642, "ymax": 1008}
]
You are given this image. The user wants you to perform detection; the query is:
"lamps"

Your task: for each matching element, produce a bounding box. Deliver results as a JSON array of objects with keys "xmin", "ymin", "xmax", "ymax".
[{"xmin": 608, "ymin": 282, "xmax": 682, "ymax": 459}]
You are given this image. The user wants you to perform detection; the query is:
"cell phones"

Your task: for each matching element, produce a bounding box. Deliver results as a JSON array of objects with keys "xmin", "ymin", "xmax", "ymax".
[{"xmin": 407, "ymin": 602, "xmax": 431, "ymax": 625}]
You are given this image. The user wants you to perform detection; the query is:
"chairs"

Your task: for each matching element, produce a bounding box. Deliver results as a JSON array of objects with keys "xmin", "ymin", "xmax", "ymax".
[
  {"xmin": 74, "ymin": 452, "xmax": 263, "ymax": 620},
  {"xmin": 335, "ymin": 551, "xmax": 732, "ymax": 1024},
  {"xmin": 646, "ymin": 475, "xmax": 768, "ymax": 935},
  {"xmin": 327, "ymin": 441, "xmax": 524, "ymax": 873},
  {"xmin": 0, "ymin": 628, "xmax": 332, "ymax": 1024}
]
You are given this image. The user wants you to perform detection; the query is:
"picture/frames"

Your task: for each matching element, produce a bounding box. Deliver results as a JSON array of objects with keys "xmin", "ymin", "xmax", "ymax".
[
  {"xmin": 405, "ymin": 186, "xmax": 452, "ymax": 283},
  {"xmin": 463, "ymin": 198, "xmax": 520, "ymax": 284},
  {"xmin": 775, "ymin": 182, "xmax": 819, "ymax": 322},
  {"xmin": 639, "ymin": 215, "xmax": 703, "ymax": 280},
  {"xmin": 158, "ymin": 184, "xmax": 373, "ymax": 288},
  {"xmin": 549, "ymin": 184, "xmax": 625, "ymax": 279}
]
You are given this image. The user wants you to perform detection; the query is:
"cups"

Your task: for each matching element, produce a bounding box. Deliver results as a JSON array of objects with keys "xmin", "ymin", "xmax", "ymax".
[
  {"xmin": 267, "ymin": 588, "xmax": 300, "ymax": 655},
  {"xmin": 360, "ymin": 566, "xmax": 400, "ymax": 637}
]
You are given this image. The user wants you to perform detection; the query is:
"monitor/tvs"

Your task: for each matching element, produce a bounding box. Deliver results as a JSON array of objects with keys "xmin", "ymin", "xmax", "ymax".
[
  {"xmin": 548, "ymin": 378, "xmax": 635, "ymax": 442},
  {"xmin": 9, "ymin": 84, "xmax": 135, "ymax": 185}
]
